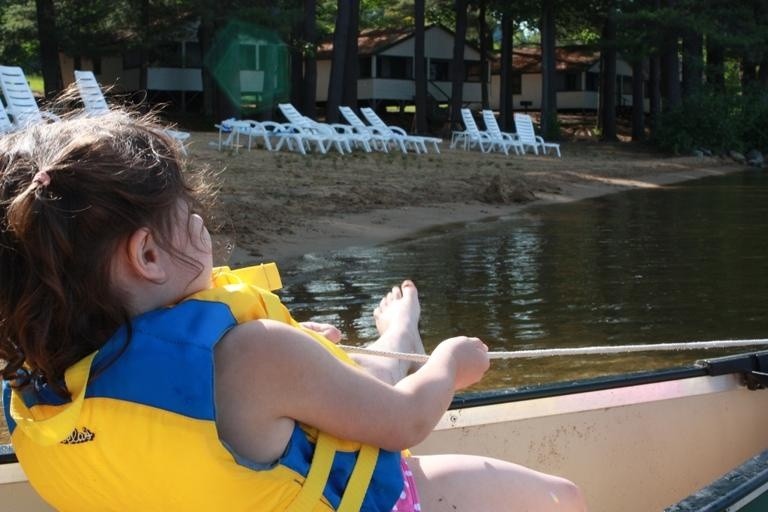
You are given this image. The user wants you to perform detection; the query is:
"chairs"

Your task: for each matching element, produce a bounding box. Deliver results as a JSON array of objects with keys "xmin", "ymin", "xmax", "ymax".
[
  {"xmin": 0, "ymin": 65, "xmax": 190, "ymax": 155},
  {"xmin": 216, "ymin": 103, "xmax": 443, "ymax": 154},
  {"xmin": 451, "ymin": 107, "xmax": 561, "ymax": 154}
]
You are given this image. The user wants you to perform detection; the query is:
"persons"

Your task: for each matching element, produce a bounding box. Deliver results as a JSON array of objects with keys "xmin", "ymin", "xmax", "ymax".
[{"xmin": 0, "ymin": 110, "xmax": 590, "ymax": 511}]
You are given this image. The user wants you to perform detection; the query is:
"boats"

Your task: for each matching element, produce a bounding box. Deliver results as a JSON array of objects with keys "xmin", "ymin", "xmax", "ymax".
[{"xmin": 0, "ymin": 349, "xmax": 768, "ymax": 511}]
